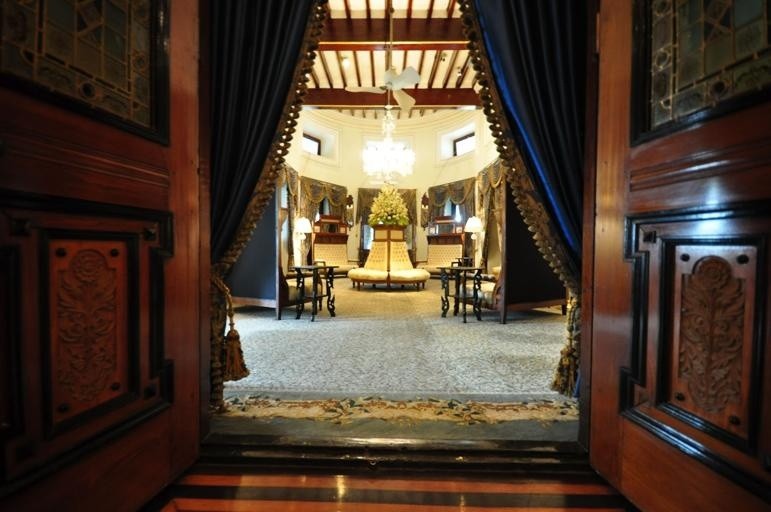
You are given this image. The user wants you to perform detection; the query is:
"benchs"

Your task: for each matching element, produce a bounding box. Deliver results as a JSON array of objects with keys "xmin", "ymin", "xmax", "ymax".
[
  {"xmin": 348, "ymin": 268, "xmax": 389, "ymax": 291},
  {"xmin": 388, "ymin": 269, "xmax": 431, "ymax": 291}
]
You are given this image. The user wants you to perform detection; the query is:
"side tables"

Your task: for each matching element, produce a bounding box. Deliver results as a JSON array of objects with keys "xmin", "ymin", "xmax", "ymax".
[
  {"xmin": 290, "ymin": 265, "xmax": 339, "ymax": 322},
  {"xmin": 436, "ymin": 267, "xmax": 485, "ymax": 323}
]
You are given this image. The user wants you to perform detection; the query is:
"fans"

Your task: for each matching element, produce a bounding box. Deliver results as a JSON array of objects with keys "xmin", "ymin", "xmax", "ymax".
[{"xmin": 344, "ymin": 0, "xmax": 422, "ymax": 113}]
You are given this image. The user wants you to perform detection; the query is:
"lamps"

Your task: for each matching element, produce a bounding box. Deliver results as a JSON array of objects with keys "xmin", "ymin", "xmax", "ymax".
[
  {"xmin": 361, "ymin": 90, "xmax": 417, "ymax": 183},
  {"xmin": 464, "ymin": 216, "xmax": 484, "ymax": 268},
  {"xmin": 295, "ymin": 217, "xmax": 313, "ymax": 266}
]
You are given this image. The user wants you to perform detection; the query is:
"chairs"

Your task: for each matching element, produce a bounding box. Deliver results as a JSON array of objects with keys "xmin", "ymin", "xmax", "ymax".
[
  {"xmin": 279, "ymin": 265, "xmax": 322, "ymax": 321},
  {"xmin": 460, "ymin": 265, "xmax": 502, "ymax": 314}
]
